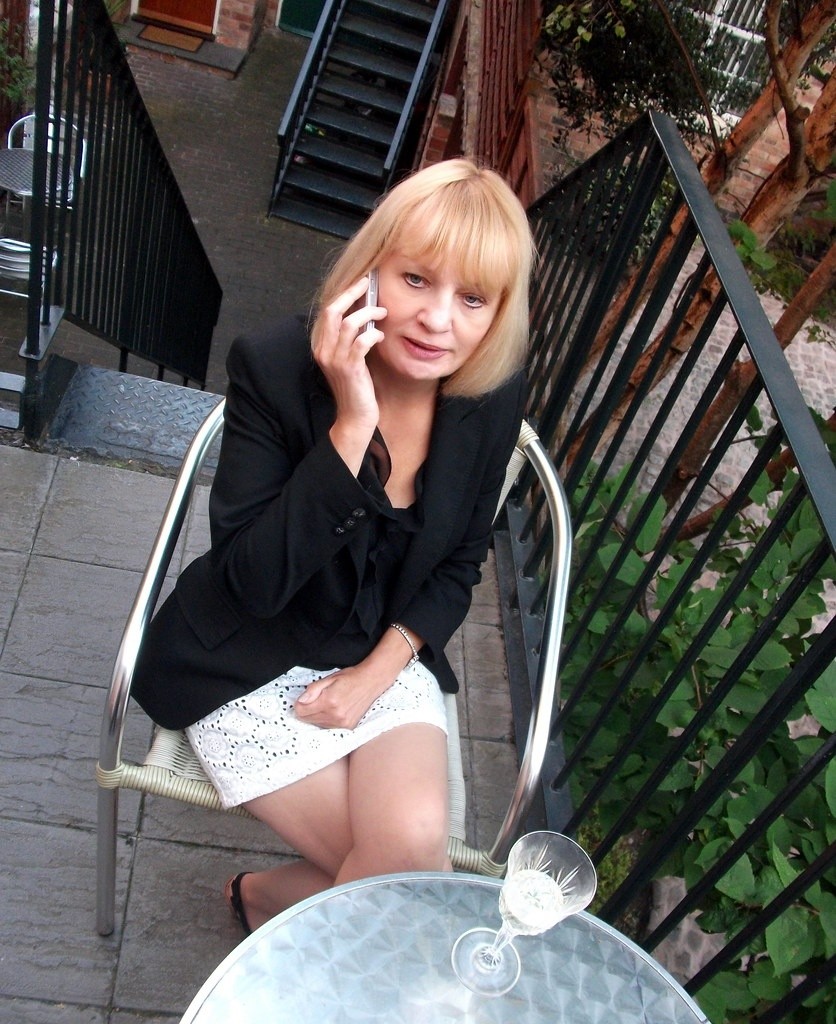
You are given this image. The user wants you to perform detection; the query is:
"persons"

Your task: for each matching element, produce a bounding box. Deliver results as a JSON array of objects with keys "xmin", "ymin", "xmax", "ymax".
[{"xmin": 137, "ymin": 161, "xmax": 531, "ymax": 933}]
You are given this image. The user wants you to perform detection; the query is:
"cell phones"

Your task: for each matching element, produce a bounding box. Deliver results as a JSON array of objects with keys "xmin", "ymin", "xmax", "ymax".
[{"xmin": 341, "ymin": 265, "xmax": 379, "ymax": 340}]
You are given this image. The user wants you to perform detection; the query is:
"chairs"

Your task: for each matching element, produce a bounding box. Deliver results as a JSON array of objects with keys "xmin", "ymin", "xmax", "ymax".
[
  {"xmin": 0, "ymin": 115, "xmax": 86, "ymax": 239},
  {"xmin": 0, "ymin": 235, "xmax": 59, "ymax": 317},
  {"xmin": 96, "ymin": 394, "xmax": 573, "ymax": 936}
]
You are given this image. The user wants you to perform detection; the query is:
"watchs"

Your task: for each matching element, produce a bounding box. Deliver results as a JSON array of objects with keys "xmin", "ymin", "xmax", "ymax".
[{"xmin": 390, "ymin": 622, "xmax": 420, "ymax": 672}]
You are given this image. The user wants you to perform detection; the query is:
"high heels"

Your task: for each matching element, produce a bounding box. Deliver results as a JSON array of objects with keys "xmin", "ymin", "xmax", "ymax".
[{"xmin": 224, "ymin": 870, "xmax": 256, "ymax": 938}]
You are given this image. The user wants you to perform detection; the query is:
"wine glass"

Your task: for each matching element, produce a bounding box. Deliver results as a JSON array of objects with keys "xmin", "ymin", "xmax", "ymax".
[{"xmin": 450, "ymin": 831, "xmax": 598, "ymax": 997}]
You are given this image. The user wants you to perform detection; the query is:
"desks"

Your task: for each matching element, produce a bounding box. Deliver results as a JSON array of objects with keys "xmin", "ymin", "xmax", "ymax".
[
  {"xmin": 0, "ymin": 149, "xmax": 74, "ymax": 241},
  {"xmin": 178, "ymin": 872, "xmax": 713, "ymax": 1024}
]
